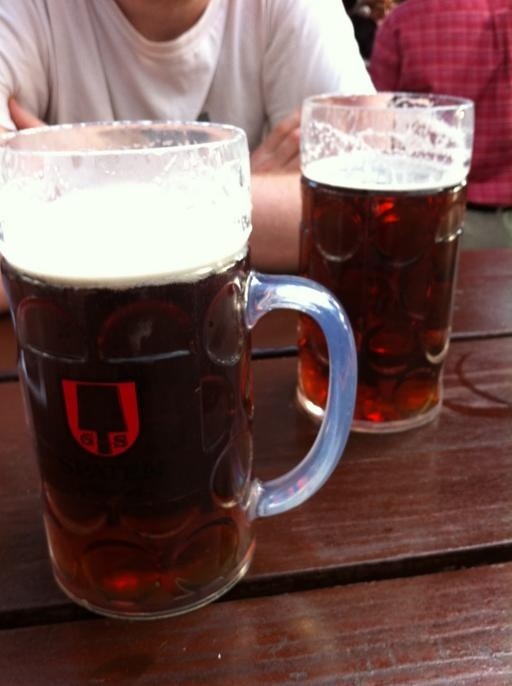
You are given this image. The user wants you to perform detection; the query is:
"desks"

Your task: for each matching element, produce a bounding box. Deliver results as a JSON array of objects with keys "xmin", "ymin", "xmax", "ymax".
[{"xmin": 1, "ymin": 247, "xmax": 511, "ymax": 685}]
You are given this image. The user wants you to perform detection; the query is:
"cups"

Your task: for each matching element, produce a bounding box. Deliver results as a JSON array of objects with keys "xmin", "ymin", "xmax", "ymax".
[
  {"xmin": 1, "ymin": 120, "xmax": 358, "ymax": 620},
  {"xmin": 299, "ymin": 94, "xmax": 475, "ymax": 433}
]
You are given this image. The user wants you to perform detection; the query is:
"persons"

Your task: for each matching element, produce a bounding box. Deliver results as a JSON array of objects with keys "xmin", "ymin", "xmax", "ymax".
[
  {"xmin": 368, "ymin": 0, "xmax": 512, "ymax": 213},
  {"xmin": 1, "ymin": 0, "xmax": 395, "ymax": 314}
]
